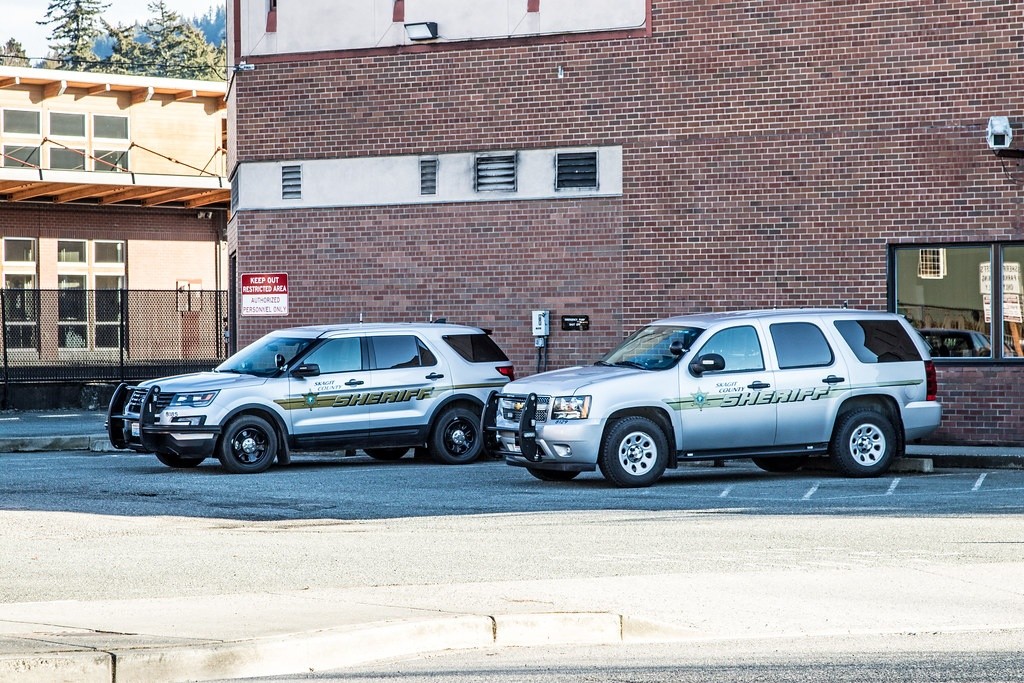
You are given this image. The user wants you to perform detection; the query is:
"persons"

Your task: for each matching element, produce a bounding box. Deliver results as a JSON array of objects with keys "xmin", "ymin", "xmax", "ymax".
[{"xmin": 933, "ymin": 337, "xmax": 949, "ymax": 357}]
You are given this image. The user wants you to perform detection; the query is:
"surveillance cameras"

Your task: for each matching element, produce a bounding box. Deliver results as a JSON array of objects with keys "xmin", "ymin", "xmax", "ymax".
[
  {"xmin": 985, "ymin": 115, "xmax": 1013, "ymax": 150},
  {"xmin": 232, "ymin": 61, "xmax": 256, "ymax": 72}
]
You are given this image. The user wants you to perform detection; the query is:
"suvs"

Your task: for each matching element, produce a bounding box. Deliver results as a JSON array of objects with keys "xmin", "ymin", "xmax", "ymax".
[
  {"xmin": 480, "ymin": 307, "xmax": 943, "ymax": 478},
  {"xmin": 105, "ymin": 317, "xmax": 514, "ymax": 474},
  {"xmin": 918, "ymin": 329, "xmax": 989, "ymax": 357}
]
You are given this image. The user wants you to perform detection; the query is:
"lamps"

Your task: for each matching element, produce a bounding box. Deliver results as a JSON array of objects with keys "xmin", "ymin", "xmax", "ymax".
[
  {"xmin": 404, "ymin": 22, "xmax": 437, "ymax": 41},
  {"xmin": 197, "ymin": 212, "xmax": 212, "ymax": 219}
]
